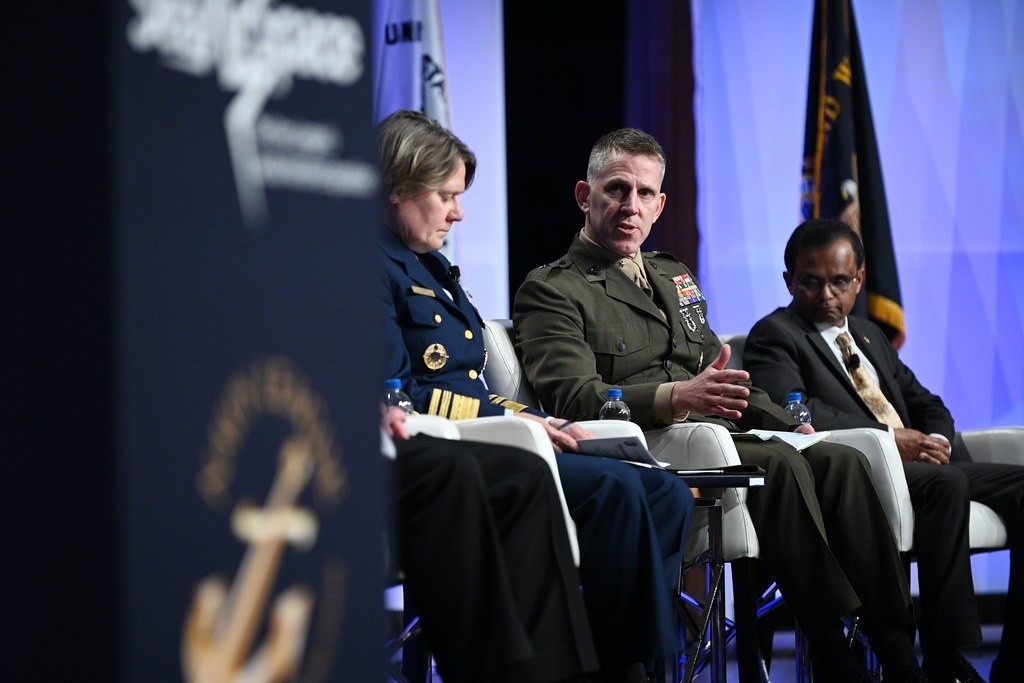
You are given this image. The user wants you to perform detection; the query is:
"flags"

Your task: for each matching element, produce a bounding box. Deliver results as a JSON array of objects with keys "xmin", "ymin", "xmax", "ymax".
[
  {"xmin": 798, "ymin": 1, "xmax": 905, "ymax": 350},
  {"xmin": 369, "ymin": 0, "xmax": 451, "ymax": 130}
]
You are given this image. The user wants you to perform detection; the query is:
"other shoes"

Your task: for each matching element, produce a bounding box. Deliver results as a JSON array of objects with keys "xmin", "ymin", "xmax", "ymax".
[
  {"xmin": 814, "ymin": 667, "xmax": 879, "ymax": 683},
  {"xmin": 923, "ymin": 655, "xmax": 986, "ymax": 683},
  {"xmin": 990, "ymin": 660, "xmax": 1024, "ymax": 683},
  {"xmin": 881, "ymin": 656, "xmax": 930, "ymax": 683}
]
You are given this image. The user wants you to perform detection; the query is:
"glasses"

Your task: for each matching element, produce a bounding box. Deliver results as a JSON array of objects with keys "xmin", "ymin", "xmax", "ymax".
[{"xmin": 791, "ymin": 273, "xmax": 857, "ymax": 294}]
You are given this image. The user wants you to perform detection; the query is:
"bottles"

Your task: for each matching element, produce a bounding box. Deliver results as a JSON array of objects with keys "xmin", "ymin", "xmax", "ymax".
[
  {"xmin": 598, "ymin": 389, "xmax": 631, "ymax": 420},
  {"xmin": 382, "ymin": 377, "xmax": 412, "ymax": 413},
  {"xmin": 785, "ymin": 393, "xmax": 812, "ymax": 425}
]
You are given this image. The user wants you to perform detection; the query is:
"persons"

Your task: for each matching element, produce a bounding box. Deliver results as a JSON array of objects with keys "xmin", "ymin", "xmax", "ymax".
[
  {"xmin": 373, "ymin": 110, "xmax": 694, "ymax": 682},
  {"xmin": 512, "ymin": 127, "xmax": 929, "ymax": 683},
  {"xmin": 742, "ymin": 218, "xmax": 1024, "ymax": 683}
]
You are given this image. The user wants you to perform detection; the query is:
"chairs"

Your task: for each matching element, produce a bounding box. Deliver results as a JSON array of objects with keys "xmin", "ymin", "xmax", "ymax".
[{"xmin": 384, "ymin": 320, "xmax": 1024, "ymax": 683}]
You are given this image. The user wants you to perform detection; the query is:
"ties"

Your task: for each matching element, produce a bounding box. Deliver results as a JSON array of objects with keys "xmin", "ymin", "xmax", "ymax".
[
  {"xmin": 836, "ymin": 332, "xmax": 905, "ymax": 430},
  {"xmin": 633, "ymin": 263, "xmax": 653, "ymax": 301}
]
